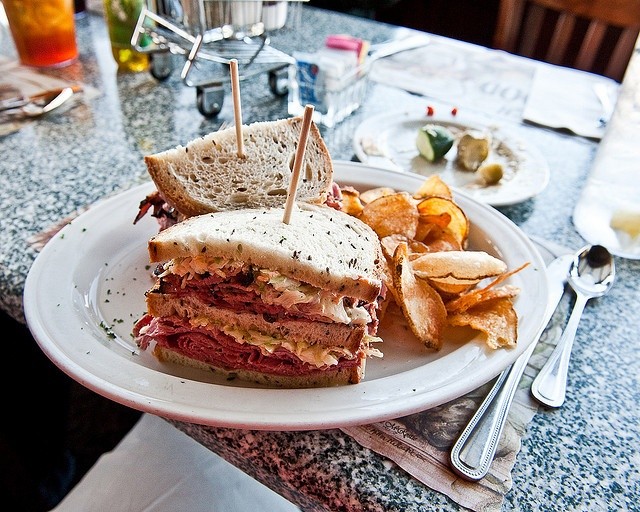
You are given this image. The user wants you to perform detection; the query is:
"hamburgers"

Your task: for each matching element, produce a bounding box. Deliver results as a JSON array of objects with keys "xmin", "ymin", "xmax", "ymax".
[
  {"xmin": 132, "ymin": 115, "xmax": 335, "ymax": 226},
  {"xmin": 128, "ymin": 202, "xmax": 390, "ymax": 388}
]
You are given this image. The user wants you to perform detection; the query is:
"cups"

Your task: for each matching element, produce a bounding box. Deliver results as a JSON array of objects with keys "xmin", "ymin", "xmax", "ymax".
[{"xmin": 2, "ymin": 0, "xmax": 79, "ymax": 73}]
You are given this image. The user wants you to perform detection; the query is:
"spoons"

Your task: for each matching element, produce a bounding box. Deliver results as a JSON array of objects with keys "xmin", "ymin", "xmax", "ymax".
[{"xmin": 530, "ymin": 245, "xmax": 616, "ymax": 408}]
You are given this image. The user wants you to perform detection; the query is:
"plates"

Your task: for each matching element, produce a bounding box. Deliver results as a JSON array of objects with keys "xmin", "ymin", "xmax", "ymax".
[
  {"xmin": 22, "ymin": 159, "xmax": 551, "ymax": 432},
  {"xmin": 352, "ymin": 105, "xmax": 547, "ymax": 208}
]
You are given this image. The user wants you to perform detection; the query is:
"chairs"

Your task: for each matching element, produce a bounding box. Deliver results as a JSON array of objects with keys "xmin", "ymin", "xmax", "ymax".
[{"xmin": 492, "ymin": 0, "xmax": 640, "ymax": 85}]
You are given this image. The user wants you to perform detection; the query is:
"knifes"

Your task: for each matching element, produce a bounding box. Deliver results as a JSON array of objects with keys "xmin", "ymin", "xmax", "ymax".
[{"xmin": 449, "ymin": 253, "xmax": 574, "ymax": 482}]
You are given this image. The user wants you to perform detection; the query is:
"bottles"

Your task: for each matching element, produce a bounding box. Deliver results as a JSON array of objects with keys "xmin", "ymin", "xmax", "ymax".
[{"xmin": 104, "ymin": 0, "xmax": 156, "ymax": 72}]
